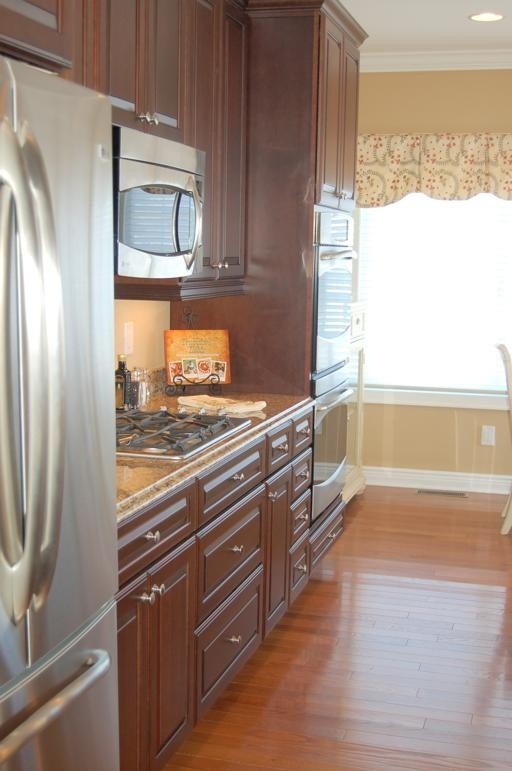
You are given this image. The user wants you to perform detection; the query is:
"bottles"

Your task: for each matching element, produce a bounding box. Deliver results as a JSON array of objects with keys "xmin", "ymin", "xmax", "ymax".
[{"xmin": 113, "ymin": 352, "xmax": 150, "ymax": 411}]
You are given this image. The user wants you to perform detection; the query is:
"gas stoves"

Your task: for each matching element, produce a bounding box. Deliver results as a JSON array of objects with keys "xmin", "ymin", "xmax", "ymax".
[{"xmin": 116, "ymin": 409, "xmax": 252, "ymax": 465}]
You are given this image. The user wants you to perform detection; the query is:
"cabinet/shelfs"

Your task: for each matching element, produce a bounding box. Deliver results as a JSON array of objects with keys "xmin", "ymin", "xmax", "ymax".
[
  {"xmin": 246, "ymin": 8, "xmax": 362, "ymax": 210},
  {"xmin": 3, "ymin": 0, "xmax": 87, "ymax": 77},
  {"xmin": 288, "ymin": 406, "xmax": 311, "ymax": 604},
  {"xmin": 185, "ymin": 2, "xmax": 250, "ymax": 296},
  {"xmin": 263, "ymin": 418, "xmax": 292, "ymax": 639},
  {"xmin": 117, "ymin": 478, "xmax": 197, "ymax": 770},
  {"xmin": 194, "ymin": 434, "xmax": 266, "ymax": 712},
  {"xmin": 87, "ymin": 2, "xmax": 198, "ymax": 146}
]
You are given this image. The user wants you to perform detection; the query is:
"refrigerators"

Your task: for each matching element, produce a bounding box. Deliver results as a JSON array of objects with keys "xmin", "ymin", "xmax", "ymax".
[{"xmin": 0, "ymin": 56, "xmax": 120, "ymax": 771}]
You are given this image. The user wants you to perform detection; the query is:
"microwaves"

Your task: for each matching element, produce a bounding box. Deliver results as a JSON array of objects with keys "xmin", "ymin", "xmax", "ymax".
[{"xmin": 111, "ymin": 122, "xmax": 206, "ymax": 280}]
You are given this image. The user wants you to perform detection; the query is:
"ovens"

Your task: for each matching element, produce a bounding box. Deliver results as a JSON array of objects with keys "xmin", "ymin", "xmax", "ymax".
[{"xmin": 312, "ymin": 211, "xmax": 358, "ymax": 525}]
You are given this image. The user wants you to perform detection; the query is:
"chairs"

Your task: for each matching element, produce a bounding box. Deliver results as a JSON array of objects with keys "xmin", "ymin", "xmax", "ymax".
[{"xmin": 496, "ymin": 344, "xmax": 512, "ymax": 535}]
